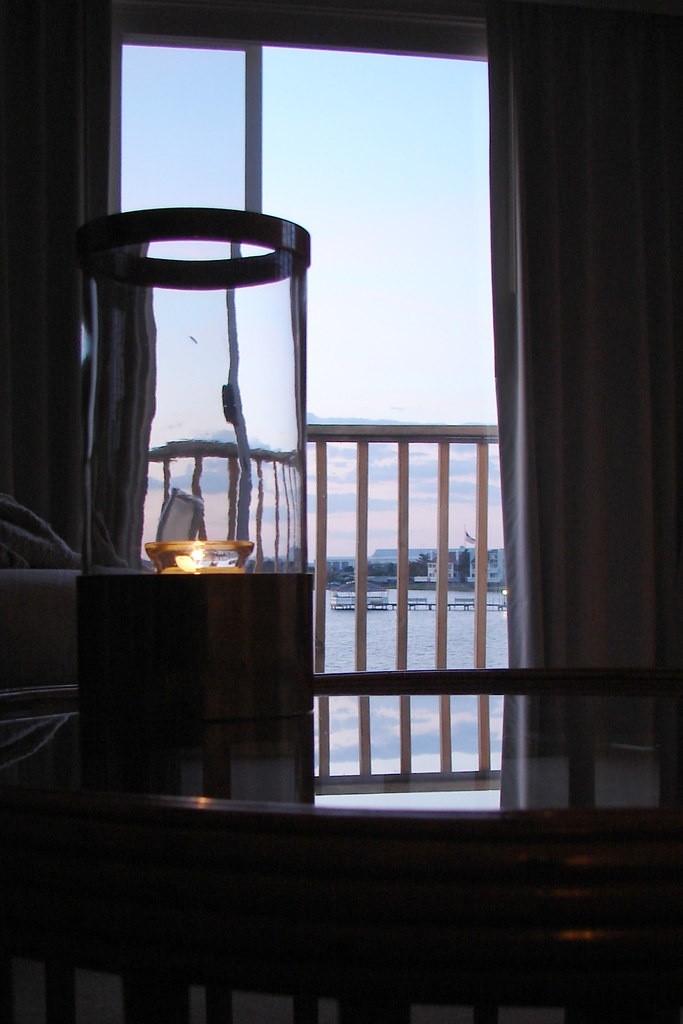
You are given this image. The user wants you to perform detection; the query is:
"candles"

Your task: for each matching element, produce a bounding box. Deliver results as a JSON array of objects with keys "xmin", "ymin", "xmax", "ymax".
[{"xmin": 146, "ymin": 540, "xmax": 251, "ymax": 573}]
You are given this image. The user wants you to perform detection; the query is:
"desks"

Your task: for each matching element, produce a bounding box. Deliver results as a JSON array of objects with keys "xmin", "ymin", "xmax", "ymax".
[{"xmin": 0, "ymin": 667, "xmax": 683, "ymax": 1024}]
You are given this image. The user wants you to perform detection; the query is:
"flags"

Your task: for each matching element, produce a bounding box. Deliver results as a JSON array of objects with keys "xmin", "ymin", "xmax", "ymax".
[{"xmin": 466, "ymin": 532, "xmax": 476, "ymax": 544}]
